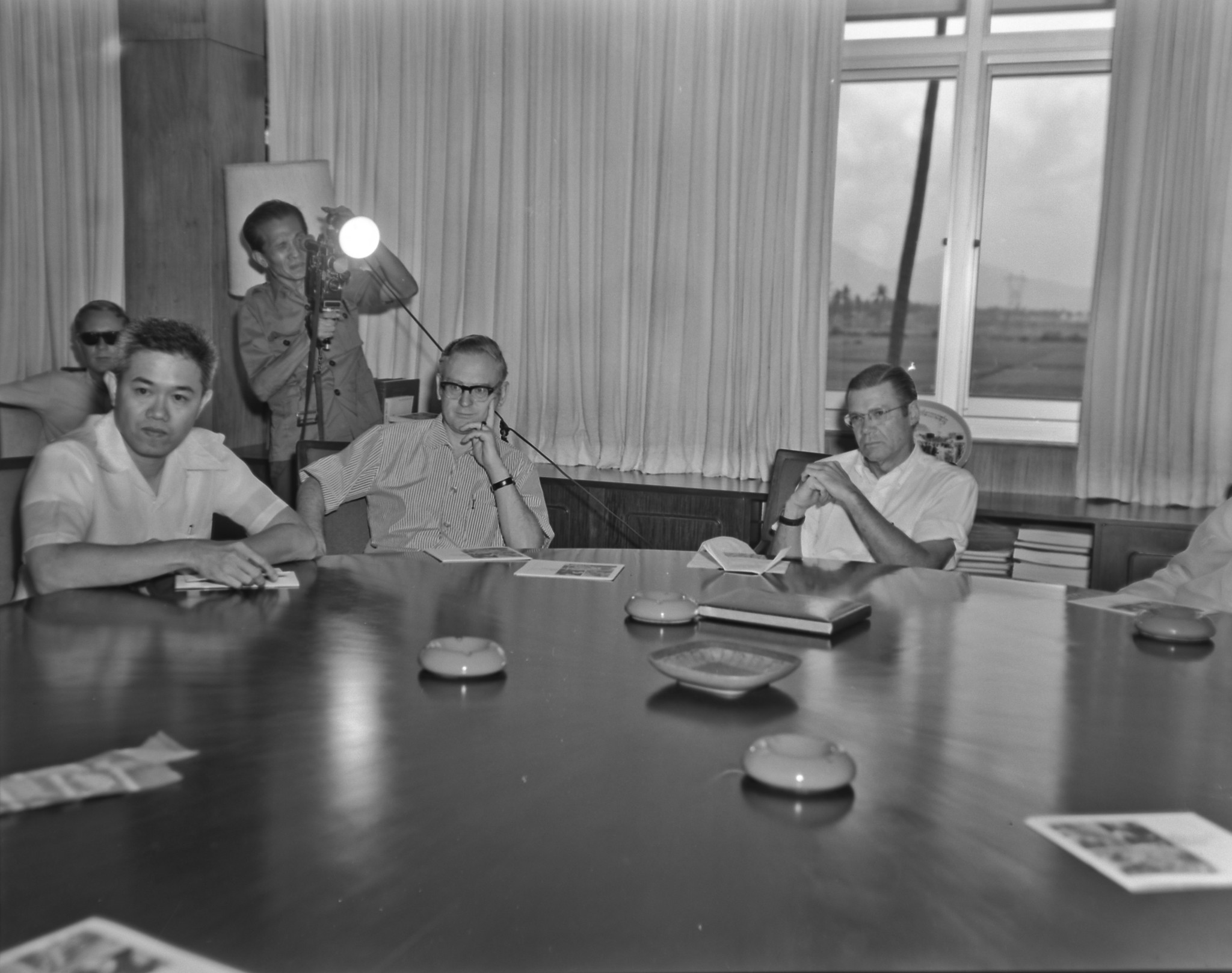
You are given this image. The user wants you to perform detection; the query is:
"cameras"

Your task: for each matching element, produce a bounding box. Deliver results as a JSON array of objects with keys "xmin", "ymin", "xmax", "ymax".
[{"xmin": 304, "ymin": 214, "xmax": 381, "ymax": 314}]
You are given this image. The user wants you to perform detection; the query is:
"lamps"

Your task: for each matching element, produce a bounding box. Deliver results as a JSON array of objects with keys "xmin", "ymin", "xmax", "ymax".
[
  {"xmin": 223, "ymin": 158, "xmax": 334, "ymax": 296},
  {"xmin": 320, "ymin": 213, "xmax": 380, "ymax": 258}
]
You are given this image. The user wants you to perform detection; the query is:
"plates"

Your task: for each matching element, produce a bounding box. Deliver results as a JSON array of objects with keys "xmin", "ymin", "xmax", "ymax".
[
  {"xmin": 913, "ymin": 399, "xmax": 972, "ymax": 467},
  {"xmin": 647, "ymin": 641, "xmax": 802, "ymax": 699}
]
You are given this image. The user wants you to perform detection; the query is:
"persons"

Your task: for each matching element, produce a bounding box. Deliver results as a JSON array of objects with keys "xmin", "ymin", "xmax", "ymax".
[
  {"xmin": 296, "ymin": 335, "xmax": 555, "ymax": 558},
  {"xmin": 0, "ymin": 283, "xmax": 321, "ymax": 604},
  {"xmin": 238, "ymin": 199, "xmax": 420, "ymax": 512},
  {"xmin": 1117, "ymin": 498, "xmax": 1232, "ymax": 611},
  {"xmin": 766, "ymin": 364, "xmax": 980, "ymax": 572}
]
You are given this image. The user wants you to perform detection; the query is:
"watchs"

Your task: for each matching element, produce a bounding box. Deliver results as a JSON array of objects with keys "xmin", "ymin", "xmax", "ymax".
[
  {"xmin": 491, "ymin": 474, "xmax": 516, "ymax": 491},
  {"xmin": 778, "ymin": 507, "xmax": 805, "ymax": 526}
]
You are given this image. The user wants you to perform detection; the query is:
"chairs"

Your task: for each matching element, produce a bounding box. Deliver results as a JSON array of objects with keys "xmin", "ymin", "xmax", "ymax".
[
  {"xmin": 297, "ymin": 439, "xmax": 371, "ymax": 553},
  {"xmin": 755, "ymin": 449, "xmax": 834, "ymax": 553}
]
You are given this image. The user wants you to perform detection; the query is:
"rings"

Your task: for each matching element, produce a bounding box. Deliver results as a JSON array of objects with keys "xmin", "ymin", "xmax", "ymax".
[{"xmin": 480, "ymin": 421, "xmax": 487, "ymax": 431}]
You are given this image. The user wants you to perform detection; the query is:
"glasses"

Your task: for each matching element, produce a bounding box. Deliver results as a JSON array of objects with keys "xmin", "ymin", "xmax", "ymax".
[
  {"xmin": 843, "ymin": 401, "xmax": 909, "ymax": 429},
  {"xmin": 440, "ymin": 372, "xmax": 503, "ymax": 403},
  {"xmin": 78, "ymin": 330, "xmax": 122, "ymax": 347}
]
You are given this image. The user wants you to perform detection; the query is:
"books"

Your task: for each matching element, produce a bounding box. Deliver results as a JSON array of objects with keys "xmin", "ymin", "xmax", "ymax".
[
  {"xmin": 954, "ymin": 522, "xmax": 1092, "ymax": 589},
  {"xmin": 697, "ymin": 586, "xmax": 872, "ymax": 635}
]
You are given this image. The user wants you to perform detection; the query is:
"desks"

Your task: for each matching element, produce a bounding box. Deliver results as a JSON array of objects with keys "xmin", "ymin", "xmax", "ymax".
[{"xmin": 0, "ymin": 550, "xmax": 1232, "ymax": 973}]
